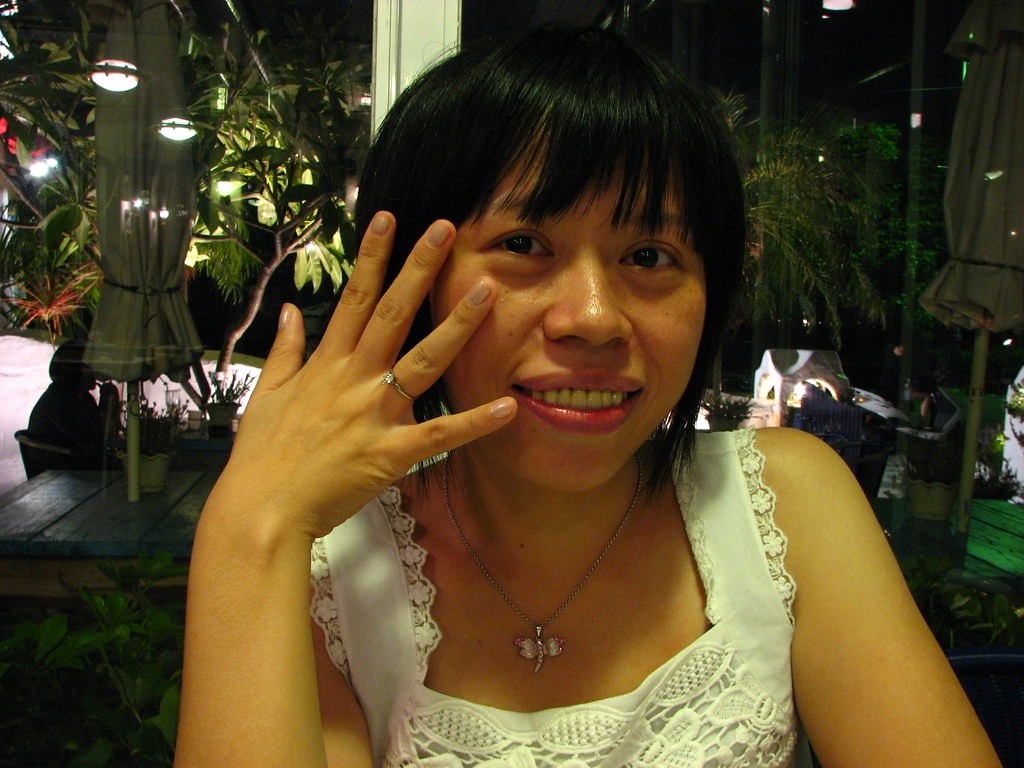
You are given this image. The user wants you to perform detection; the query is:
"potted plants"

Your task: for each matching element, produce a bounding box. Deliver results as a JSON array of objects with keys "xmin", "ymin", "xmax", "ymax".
[
  {"xmin": 114, "ymin": 403, "xmax": 181, "ymax": 494},
  {"xmin": 901, "ymin": 423, "xmax": 972, "ymax": 523},
  {"xmin": 701, "ymin": 382, "xmax": 759, "ymax": 431},
  {"xmin": 202, "ymin": 373, "xmax": 248, "ymax": 429}
]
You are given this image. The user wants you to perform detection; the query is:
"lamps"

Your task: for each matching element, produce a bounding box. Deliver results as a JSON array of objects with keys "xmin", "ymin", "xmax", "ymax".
[
  {"xmin": 154, "ymin": 117, "xmax": 206, "ymax": 141},
  {"xmin": 85, "ymin": 59, "xmax": 152, "ymax": 94}
]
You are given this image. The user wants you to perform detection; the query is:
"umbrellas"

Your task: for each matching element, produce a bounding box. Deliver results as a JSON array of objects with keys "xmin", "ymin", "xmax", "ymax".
[
  {"xmin": 80, "ymin": 0, "xmax": 207, "ymax": 501},
  {"xmin": 915, "ymin": 0, "xmax": 1024, "ymax": 534}
]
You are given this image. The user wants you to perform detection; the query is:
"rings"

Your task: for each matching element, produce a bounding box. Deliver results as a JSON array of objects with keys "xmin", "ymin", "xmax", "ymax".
[{"xmin": 381, "ymin": 368, "xmax": 414, "ymax": 400}]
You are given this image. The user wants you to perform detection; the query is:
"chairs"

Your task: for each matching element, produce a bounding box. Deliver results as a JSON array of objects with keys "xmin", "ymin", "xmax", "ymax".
[{"xmin": 16, "ymin": 431, "xmax": 93, "ymax": 483}]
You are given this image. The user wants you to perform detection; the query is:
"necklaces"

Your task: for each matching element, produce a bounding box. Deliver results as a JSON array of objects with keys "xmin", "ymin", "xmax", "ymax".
[{"xmin": 443, "ymin": 456, "xmax": 644, "ymax": 672}]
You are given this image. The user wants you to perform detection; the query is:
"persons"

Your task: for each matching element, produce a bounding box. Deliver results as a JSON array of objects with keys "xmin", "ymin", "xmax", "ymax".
[
  {"xmin": 881, "ymin": 334, "xmax": 943, "ymax": 432},
  {"xmin": 172, "ymin": 36, "xmax": 1002, "ymax": 768},
  {"xmin": 28, "ymin": 338, "xmax": 123, "ymax": 473}
]
